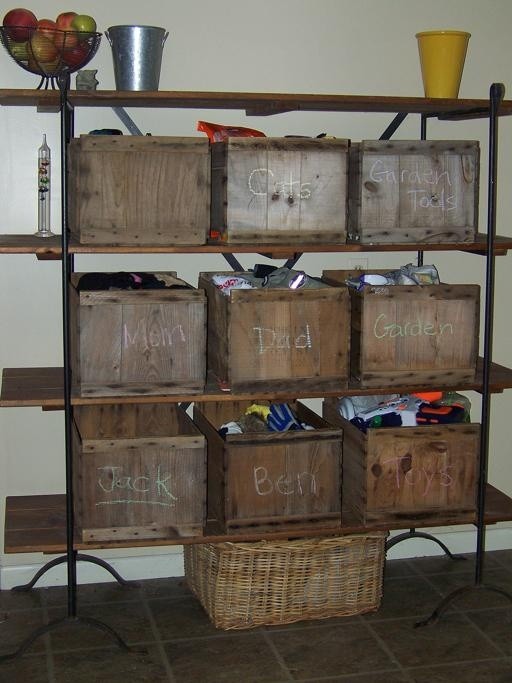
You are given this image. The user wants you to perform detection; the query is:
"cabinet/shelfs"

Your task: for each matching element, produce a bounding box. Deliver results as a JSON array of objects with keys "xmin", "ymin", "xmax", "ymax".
[{"xmin": 0, "ymin": 70, "xmax": 512, "ymax": 659}]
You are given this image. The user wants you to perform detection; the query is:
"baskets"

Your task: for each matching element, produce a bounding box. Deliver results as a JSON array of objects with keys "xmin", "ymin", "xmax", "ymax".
[
  {"xmin": 0, "ymin": 25, "xmax": 102, "ymax": 78},
  {"xmin": 183, "ymin": 530, "xmax": 390, "ymax": 631}
]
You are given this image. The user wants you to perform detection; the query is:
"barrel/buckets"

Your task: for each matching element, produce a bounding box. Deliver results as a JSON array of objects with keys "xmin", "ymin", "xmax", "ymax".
[{"xmin": 104, "ymin": 22, "xmax": 171, "ymax": 92}]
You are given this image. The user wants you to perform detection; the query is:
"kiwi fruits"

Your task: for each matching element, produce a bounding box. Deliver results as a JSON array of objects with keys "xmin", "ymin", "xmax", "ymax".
[{"xmin": 25, "ymin": 33, "xmax": 59, "ymax": 72}]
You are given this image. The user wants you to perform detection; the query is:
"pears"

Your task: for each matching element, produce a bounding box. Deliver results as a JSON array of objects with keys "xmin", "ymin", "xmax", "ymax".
[{"xmin": 8, "ymin": 39, "xmax": 29, "ymax": 61}]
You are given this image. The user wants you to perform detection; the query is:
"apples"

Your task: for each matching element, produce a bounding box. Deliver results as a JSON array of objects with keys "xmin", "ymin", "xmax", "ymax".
[
  {"xmin": 3, "ymin": 9, "xmax": 37, "ymax": 43},
  {"xmin": 38, "ymin": 12, "xmax": 97, "ymax": 68}
]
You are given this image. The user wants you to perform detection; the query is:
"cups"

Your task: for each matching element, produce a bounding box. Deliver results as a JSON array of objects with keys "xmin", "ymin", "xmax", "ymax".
[{"xmin": 414, "ymin": 27, "xmax": 471, "ymax": 99}]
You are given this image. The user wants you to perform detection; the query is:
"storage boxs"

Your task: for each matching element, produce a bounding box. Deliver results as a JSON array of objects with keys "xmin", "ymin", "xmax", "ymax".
[
  {"xmin": 213, "ymin": 136, "xmax": 357, "ymax": 244},
  {"xmin": 195, "ymin": 266, "xmax": 355, "ymax": 387},
  {"xmin": 65, "ymin": 131, "xmax": 211, "ymax": 246},
  {"xmin": 188, "ymin": 401, "xmax": 343, "ymax": 532},
  {"xmin": 72, "ymin": 404, "xmax": 210, "ymax": 541},
  {"xmin": 321, "ymin": 397, "xmax": 481, "ymax": 524},
  {"xmin": 322, "ymin": 268, "xmax": 481, "ymax": 388},
  {"xmin": 73, "ymin": 269, "xmax": 211, "ymax": 397},
  {"xmin": 343, "ymin": 142, "xmax": 478, "ymax": 244}
]
